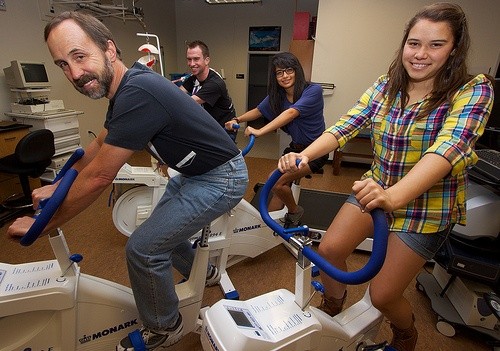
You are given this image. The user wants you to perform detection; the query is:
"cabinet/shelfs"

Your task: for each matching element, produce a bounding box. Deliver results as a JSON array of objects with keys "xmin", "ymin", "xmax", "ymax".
[{"xmin": 4, "ymin": 108, "xmax": 85, "ymax": 182}]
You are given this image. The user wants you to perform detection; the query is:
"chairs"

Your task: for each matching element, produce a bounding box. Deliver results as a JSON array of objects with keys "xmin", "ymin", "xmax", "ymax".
[{"xmin": 0, "ymin": 129, "xmax": 56, "ymax": 229}]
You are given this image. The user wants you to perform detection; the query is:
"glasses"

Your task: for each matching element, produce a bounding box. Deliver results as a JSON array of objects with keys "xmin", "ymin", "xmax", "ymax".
[{"xmin": 275, "ymin": 67, "xmax": 295, "ymax": 76}]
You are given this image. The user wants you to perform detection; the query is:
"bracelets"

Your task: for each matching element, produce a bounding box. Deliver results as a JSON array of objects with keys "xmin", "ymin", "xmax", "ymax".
[{"xmin": 228, "ymin": 114, "xmax": 244, "ymax": 127}]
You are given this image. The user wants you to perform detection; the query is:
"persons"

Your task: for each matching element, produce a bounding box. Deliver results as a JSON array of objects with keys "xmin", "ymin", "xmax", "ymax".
[
  {"xmin": 278, "ymin": 1, "xmax": 500, "ymax": 351},
  {"xmin": 178, "ymin": 40, "xmax": 236, "ymax": 178},
  {"xmin": 226, "ymin": 52, "xmax": 331, "ymax": 236},
  {"xmin": 9, "ymin": 11, "xmax": 250, "ymax": 351}
]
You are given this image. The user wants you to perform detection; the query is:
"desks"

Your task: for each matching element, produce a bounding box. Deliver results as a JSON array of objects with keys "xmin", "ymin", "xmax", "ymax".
[{"xmin": 0, "ymin": 123, "xmax": 42, "ymax": 191}]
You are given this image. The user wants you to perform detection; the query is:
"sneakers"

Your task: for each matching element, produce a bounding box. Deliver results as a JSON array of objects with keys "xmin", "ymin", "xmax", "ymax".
[
  {"xmin": 284, "ymin": 206, "xmax": 304, "ymax": 228},
  {"xmin": 177, "ymin": 264, "xmax": 220, "ymax": 287},
  {"xmin": 118, "ymin": 312, "xmax": 184, "ymax": 351}
]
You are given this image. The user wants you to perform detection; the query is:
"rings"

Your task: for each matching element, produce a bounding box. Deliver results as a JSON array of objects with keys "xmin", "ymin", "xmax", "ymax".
[{"xmin": 283, "ymin": 158, "xmax": 292, "ymax": 167}]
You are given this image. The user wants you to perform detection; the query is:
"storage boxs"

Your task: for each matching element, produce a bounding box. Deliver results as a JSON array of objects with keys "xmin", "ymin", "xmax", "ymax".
[{"xmin": 293, "ymin": 12, "xmax": 310, "ymax": 40}]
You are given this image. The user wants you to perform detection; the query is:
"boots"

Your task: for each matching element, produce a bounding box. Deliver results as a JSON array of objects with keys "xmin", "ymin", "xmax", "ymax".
[
  {"xmin": 385, "ymin": 312, "xmax": 418, "ymax": 351},
  {"xmin": 316, "ymin": 290, "xmax": 347, "ymax": 317}
]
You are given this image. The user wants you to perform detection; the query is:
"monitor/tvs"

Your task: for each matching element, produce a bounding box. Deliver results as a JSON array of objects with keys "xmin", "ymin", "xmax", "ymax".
[{"xmin": 3, "ymin": 60, "xmax": 51, "ymax": 89}]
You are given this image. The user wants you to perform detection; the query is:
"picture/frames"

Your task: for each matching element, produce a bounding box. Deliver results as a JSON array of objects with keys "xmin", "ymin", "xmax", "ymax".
[{"xmin": 247, "ymin": 24, "xmax": 282, "ymax": 54}]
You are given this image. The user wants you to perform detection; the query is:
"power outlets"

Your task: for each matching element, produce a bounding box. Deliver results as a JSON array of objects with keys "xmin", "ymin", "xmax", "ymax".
[
  {"xmin": 87, "ymin": 128, "xmax": 93, "ymax": 136},
  {"xmin": 235, "ymin": 73, "xmax": 245, "ymax": 80},
  {"xmin": 10, "ymin": 117, "xmax": 16, "ymax": 122}
]
